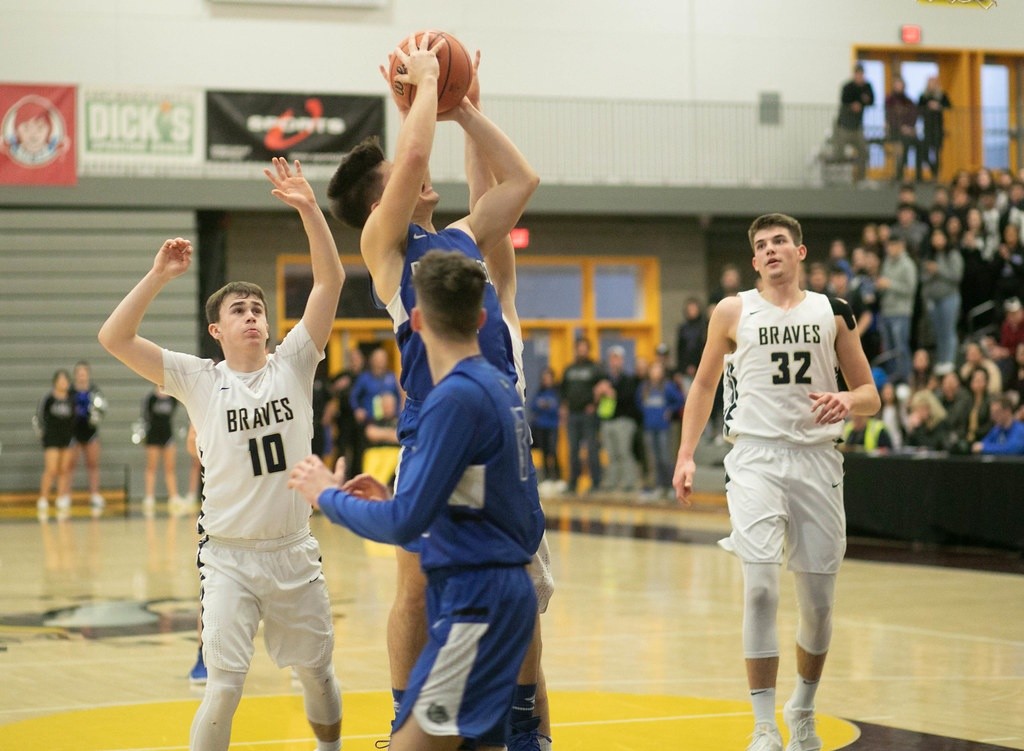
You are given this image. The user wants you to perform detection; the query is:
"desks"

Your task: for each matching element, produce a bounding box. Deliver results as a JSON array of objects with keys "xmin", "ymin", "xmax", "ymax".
[{"xmin": 841, "ymin": 445, "xmax": 1024, "ymax": 555}]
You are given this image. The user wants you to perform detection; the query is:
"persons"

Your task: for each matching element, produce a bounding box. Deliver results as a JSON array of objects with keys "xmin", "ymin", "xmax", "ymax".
[
  {"xmin": 35, "ymin": 361, "xmax": 105, "ymax": 508},
  {"xmin": 533, "ymin": 263, "xmax": 742, "ymax": 502},
  {"xmin": 672, "ymin": 213, "xmax": 881, "ymax": 751},
  {"xmin": 288, "ymin": 249, "xmax": 546, "ymax": 751},
  {"xmin": 97, "ymin": 157, "xmax": 346, "ymax": 751},
  {"xmin": 799, "ymin": 167, "xmax": 1024, "ymax": 455},
  {"xmin": 326, "ymin": 31, "xmax": 556, "ymax": 751},
  {"xmin": 834, "ymin": 64, "xmax": 952, "ymax": 181},
  {"xmin": 314, "ymin": 347, "xmax": 404, "ymax": 481},
  {"xmin": 143, "ymin": 385, "xmax": 182, "ymax": 517}
]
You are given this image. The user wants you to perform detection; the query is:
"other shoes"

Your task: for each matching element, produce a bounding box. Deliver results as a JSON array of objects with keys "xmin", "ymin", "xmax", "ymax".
[
  {"xmin": 89, "ymin": 491, "xmax": 103, "ymax": 506},
  {"xmin": 53, "ymin": 494, "xmax": 71, "ymax": 510},
  {"xmin": 187, "ymin": 494, "xmax": 198, "ymax": 510},
  {"xmin": 168, "ymin": 496, "xmax": 185, "ymax": 509},
  {"xmin": 36, "ymin": 497, "xmax": 49, "ymax": 511},
  {"xmin": 144, "ymin": 495, "xmax": 154, "ymax": 508}
]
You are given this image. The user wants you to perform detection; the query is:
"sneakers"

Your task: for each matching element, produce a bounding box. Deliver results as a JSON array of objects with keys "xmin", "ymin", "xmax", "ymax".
[
  {"xmin": 189, "ymin": 649, "xmax": 208, "ymax": 684},
  {"xmin": 745, "ymin": 724, "xmax": 783, "ymax": 751},
  {"xmin": 375, "ymin": 720, "xmax": 394, "ymax": 749},
  {"xmin": 783, "ymin": 698, "xmax": 821, "ymax": 751},
  {"xmin": 503, "ymin": 711, "xmax": 552, "ymax": 751}
]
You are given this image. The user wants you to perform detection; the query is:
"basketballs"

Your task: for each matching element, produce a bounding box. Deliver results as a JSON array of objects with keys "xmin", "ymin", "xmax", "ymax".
[{"xmin": 388, "ymin": 32, "xmax": 472, "ymax": 116}]
